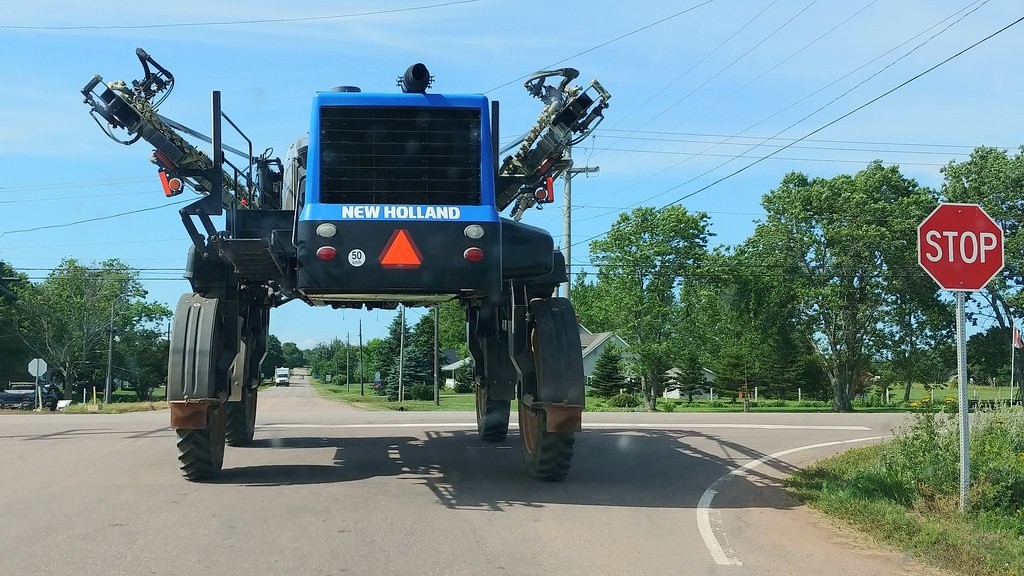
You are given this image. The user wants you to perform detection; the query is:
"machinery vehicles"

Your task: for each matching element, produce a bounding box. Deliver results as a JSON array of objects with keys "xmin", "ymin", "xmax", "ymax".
[{"xmin": 79, "ymin": 46, "xmax": 614, "ymax": 485}]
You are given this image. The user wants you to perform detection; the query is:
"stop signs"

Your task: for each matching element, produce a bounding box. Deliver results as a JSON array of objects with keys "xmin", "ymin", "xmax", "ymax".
[{"xmin": 917, "ymin": 203, "xmax": 1005, "ymax": 292}]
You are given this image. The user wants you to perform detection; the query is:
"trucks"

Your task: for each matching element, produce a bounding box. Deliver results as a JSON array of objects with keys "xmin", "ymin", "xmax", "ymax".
[{"xmin": 274, "ymin": 366, "xmax": 292, "ymax": 387}]
